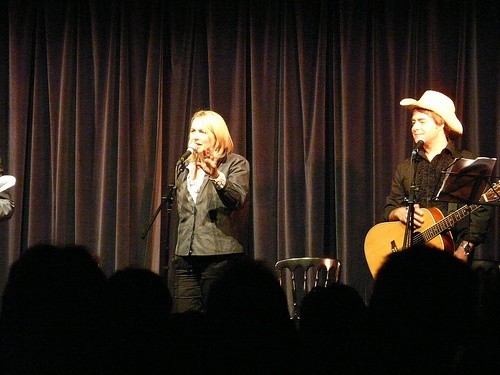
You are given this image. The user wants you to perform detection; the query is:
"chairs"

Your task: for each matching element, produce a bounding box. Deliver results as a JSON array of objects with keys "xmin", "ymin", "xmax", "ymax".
[{"xmin": 274, "ymin": 257, "xmax": 342, "ymax": 320}]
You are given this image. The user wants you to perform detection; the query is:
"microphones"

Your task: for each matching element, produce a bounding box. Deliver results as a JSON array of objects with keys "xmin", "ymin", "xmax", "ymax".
[
  {"xmin": 178, "ymin": 143, "xmax": 198, "ymax": 166},
  {"xmin": 412, "ymin": 137, "xmax": 427, "ymax": 157}
]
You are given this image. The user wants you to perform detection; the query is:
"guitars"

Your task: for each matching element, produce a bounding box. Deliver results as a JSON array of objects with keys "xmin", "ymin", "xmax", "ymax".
[{"xmin": 364, "ymin": 180, "xmax": 500, "ymax": 280}]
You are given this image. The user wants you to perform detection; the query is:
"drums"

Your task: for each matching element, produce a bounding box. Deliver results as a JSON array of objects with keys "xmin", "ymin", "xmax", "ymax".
[{"xmin": 274, "ymin": 258, "xmax": 341, "ymax": 321}]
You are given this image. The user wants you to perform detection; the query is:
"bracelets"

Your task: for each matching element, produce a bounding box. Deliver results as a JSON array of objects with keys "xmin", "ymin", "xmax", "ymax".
[{"xmin": 459, "ymin": 242, "xmax": 473, "ymax": 256}]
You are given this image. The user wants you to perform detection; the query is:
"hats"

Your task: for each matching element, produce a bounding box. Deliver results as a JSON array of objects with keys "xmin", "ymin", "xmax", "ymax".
[{"xmin": 399, "ymin": 90, "xmax": 463, "ymax": 134}]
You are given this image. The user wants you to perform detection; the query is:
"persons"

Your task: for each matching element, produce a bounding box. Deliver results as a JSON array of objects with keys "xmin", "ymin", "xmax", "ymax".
[
  {"xmin": 1, "ymin": 242, "xmax": 500, "ymax": 375},
  {"xmin": 384, "ymin": 90, "xmax": 494, "ymax": 267},
  {"xmin": 174, "ymin": 110, "xmax": 251, "ymax": 317},
  {"xmin": 0, "ymin": 160, "xmax": 16, "ymax": 224}
]
